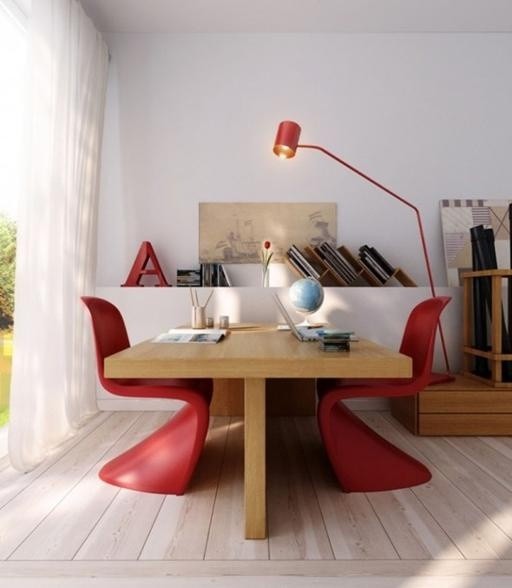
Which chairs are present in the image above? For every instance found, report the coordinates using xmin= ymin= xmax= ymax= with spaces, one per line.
xmin=316 ymin=294 xmax=452 ymax=494
xmin=80 ymin=295 xmax=213 ymax=498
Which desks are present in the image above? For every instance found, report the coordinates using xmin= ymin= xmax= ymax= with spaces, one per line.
xmin=103 ymin=320 xmax=413 ymax=541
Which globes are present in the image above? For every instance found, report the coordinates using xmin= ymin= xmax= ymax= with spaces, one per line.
xmin=289 ymin=276 xmax=324 ymax=327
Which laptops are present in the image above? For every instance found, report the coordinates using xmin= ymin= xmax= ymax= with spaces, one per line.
xmin=273 ymin=292 xmax=358 ymax=343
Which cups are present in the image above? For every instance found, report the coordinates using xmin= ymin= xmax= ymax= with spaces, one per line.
xmin=192 ymin=306 xmax=214 ymax=328
xmin=219 ymin=315 xmax=229 ymax=329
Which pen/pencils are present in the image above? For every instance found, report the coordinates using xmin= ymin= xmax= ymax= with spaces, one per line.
xmin=189 ymin=287 xmax=214 ymax=308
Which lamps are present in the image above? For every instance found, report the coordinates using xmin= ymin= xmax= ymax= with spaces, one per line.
xmin=272 ymin=119 xmax=451 ymax=374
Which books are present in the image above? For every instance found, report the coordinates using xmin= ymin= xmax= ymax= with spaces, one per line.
xmin=150 ymin=327 xmax=232 ymax=345
xmin=176 ymin=262 xmax=231 ymax=286
xmin=287 ymin=241 xmax=394 ymax=284
